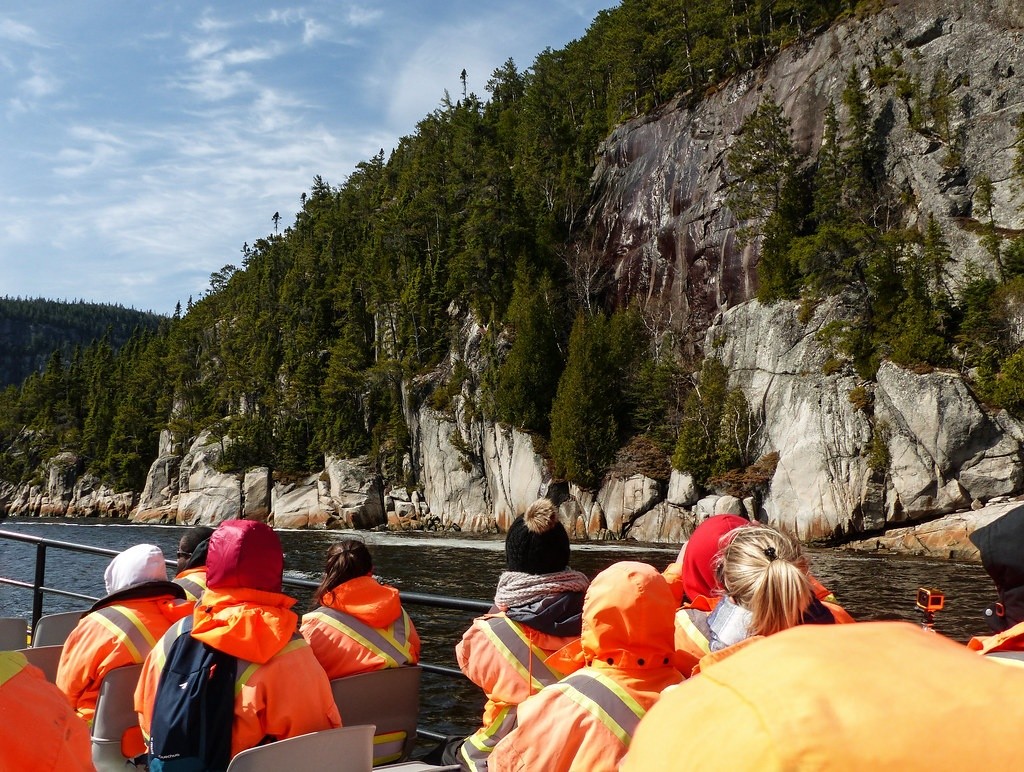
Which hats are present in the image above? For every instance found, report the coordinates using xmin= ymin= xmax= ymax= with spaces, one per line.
xmin=506 ymin=498 xmax=570 ymax=573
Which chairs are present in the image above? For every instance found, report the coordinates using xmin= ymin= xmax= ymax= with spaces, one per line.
xmin=0 ymin=617 xmax=28 ymax=651
xmin=15 ymin=644 xmax=64 ymax=683
xmin=330 ymin=666 xmax=423 ymax=763
xmin=31 ymin=609 xmax=89 ymax=648
xmin=90 ymin=663 xmax=147 ymax=772
xmin=226 ymin=724 xmax=377 ymax=772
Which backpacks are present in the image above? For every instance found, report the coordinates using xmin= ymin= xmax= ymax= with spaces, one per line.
xmin=133 ymin=632 xmax=237 ymax=772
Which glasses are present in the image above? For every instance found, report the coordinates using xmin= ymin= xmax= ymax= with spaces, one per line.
xmin=176 ymin=549 xmax=191 ymax=558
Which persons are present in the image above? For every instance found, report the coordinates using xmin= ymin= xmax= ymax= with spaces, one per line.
xmin=298 ymin=539 xmax=420 ymax=769
xmin=617 ymin=622 xmax=1024 ymax=772
xmin=421 ymin=497 xmax=590 ymax=772
xmin=661 ymin=514 xmax=856 ymax=671
xmin=964 ymin=505 xmax=1024 ymax=656
xmin=171 ymin=526 xmax=214 ymax=604
xmin=487 ymin=561 xmax=688 ymax=772
xmin=123 ymin=520 xmax=342 ymax=772
xmin=55 ymin=543 xmax=194 ymax=733
